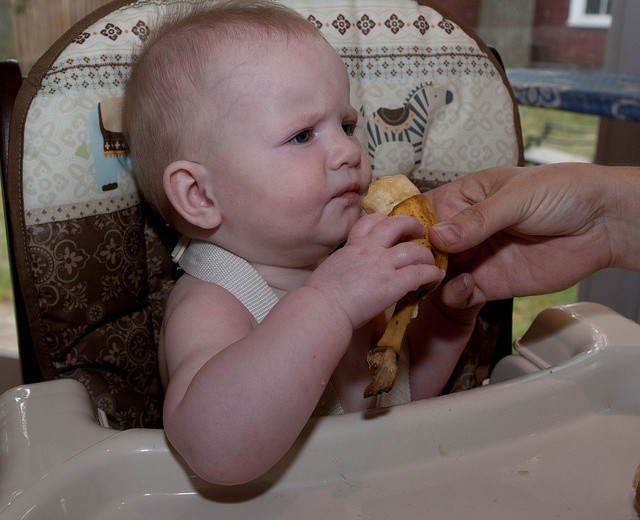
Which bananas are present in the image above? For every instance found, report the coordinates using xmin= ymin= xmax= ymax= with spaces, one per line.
xmin=361 ymin=174 xmax=448 ymax=400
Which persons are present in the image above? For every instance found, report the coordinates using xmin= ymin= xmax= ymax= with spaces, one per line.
xmin=123 ymin=4 xmax=529 ymax=486
xmin=425 ymin=162 xmax=640 ymax=312
